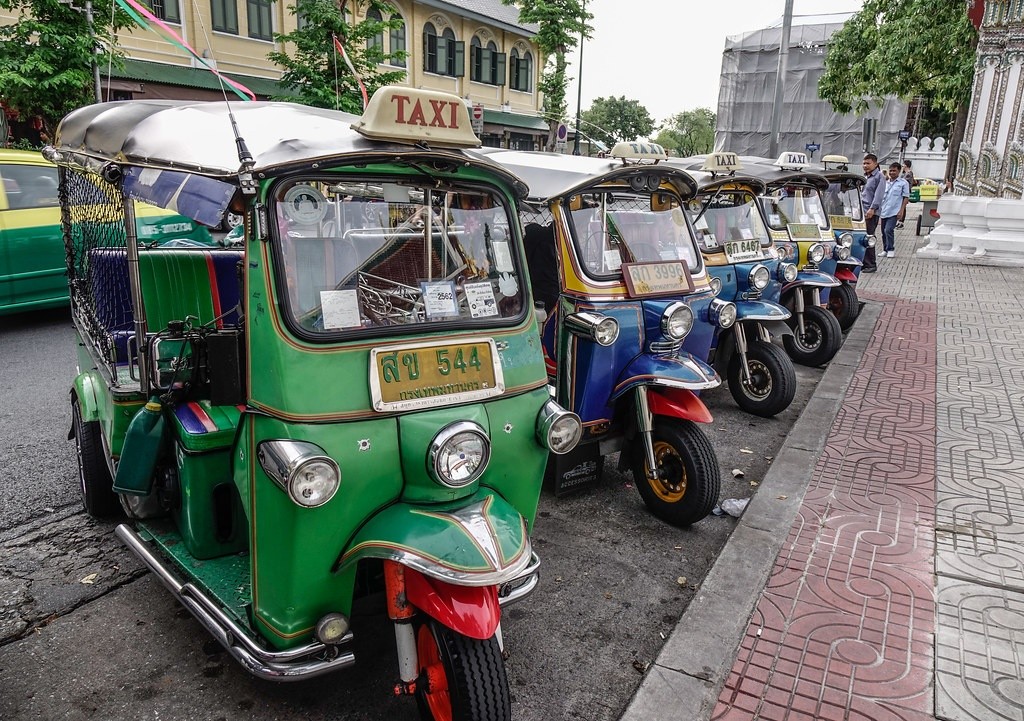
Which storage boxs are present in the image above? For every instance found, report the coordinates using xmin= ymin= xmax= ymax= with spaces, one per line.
xmin=911 ymin=185 xmax=939 ymax=199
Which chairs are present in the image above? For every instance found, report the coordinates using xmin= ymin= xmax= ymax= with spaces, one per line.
xmin=15 ymin=176 xmax=60 ymax=207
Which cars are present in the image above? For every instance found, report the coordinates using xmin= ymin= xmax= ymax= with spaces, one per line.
xmin=0 ymin=147 xmax=214 ymax=319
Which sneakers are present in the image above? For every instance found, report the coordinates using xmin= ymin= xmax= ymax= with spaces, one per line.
xmin=887 ymin=251 xmax=894 ymax=258
xmin=861 ymin=267 xmax=877 ymax=273
xmin=878 ymin=251 xmax=887 ymax=256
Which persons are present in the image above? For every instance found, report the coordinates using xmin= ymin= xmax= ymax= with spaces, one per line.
xmin=860 ymin=154 xmax=913 ymax=273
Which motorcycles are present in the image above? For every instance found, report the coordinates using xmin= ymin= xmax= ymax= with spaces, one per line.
xmin=460 ymin=140 xmax=879 ymax=531
xmin=41 ymin=84 xmax=585 ymax=721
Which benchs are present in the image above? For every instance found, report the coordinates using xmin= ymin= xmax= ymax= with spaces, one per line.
xmin=591 ymin=209 xmax=676 ymax=242
xmin=87 ymin=245 xmax=246 ymax=452
xmin=343 ymin=227 xmax=506 ymax=272
